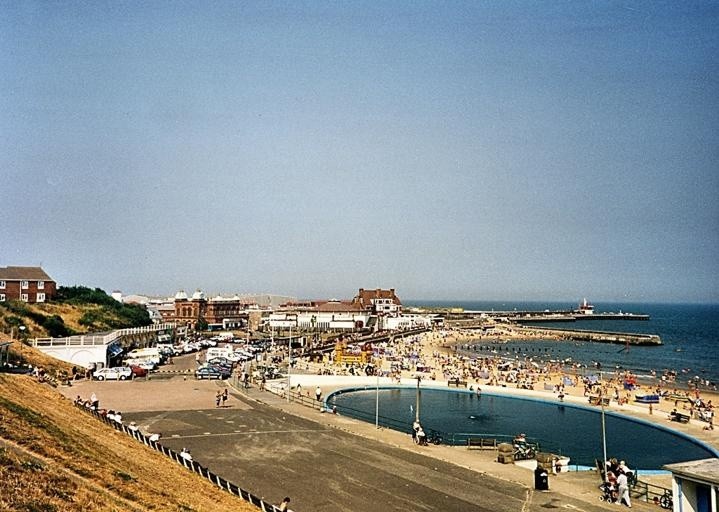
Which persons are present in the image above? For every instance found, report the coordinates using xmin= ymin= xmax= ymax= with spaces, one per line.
xmin=74 ymin=391 xmax=194 ymax=471
xmin=30 ymin=363 xmax=98 ymax=386
xmin=281 ymin=496 xmax=293 ymax=511
xmin=598 ymin=456 xmax=634 ymax=507
xmin=195 ymin=326 xmax=719 ymax=458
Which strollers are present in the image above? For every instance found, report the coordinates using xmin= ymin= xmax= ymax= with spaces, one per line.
xmin=599 ymin=470 xmax=620 ymax=503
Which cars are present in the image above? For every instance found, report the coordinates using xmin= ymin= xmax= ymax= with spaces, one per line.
xmin=89 ymin=331 xmax=272 ymax=382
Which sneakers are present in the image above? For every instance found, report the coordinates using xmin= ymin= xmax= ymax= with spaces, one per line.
xmin=628 ymin=503 xmax=632 ymax=508
xmin=614 ymin=501 xmax=622 ymax=506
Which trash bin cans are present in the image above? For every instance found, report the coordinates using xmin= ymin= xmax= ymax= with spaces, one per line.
xmin=535 ymin=465 xmax=548 ymax=490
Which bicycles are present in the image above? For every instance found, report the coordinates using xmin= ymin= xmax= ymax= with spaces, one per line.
xmin=660 ymin=489 xmax=672 ymax=508
xmin=426 ymin=430 xmax=443 ymax=445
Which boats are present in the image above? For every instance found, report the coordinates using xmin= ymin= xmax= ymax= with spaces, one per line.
xmin=665 ymin=394 xmax=690 ymax=403
xmin=634 ymin=393 xmax=660 ymax=403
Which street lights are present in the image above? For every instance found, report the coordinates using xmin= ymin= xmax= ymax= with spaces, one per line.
xmin=412 ymin=371 xmax=426 ymax=425
xmin=589 ymin=371 xmax=608 ymax=478
xmin=19 ymin=326 xmax=26 ymax=362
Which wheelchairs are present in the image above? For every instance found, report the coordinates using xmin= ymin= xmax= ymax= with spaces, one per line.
xmin=416 ymin=430 xmax=427 ymax=446
